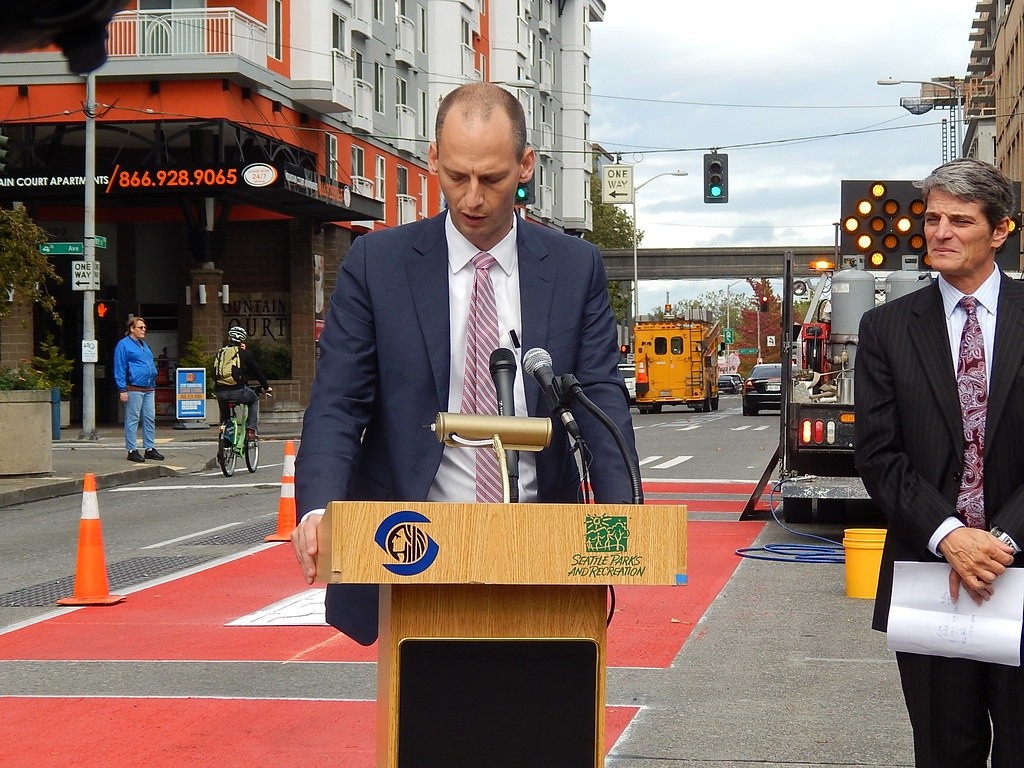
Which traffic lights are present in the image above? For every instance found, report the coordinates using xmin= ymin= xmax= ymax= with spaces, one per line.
xmin=515 ymin=171 xmax=535 ymax=205
xmin=704 ymin=153 xmax=728 ymax=204
xmin=665 ymin=304 xmax=672 ymax=316
xmin=759 ymin=294 xmax=769 ymax=314
xmin=94 ymin=300 xmax=110 ymax=323
xmin=621 ymin=345 xmax=630 ymax=354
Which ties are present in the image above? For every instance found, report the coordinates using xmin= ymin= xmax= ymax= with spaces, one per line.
xmin=460 ymin=253 xmax=504 ymax=503
xmin=956 ymin=296 xmax=987 ymax=530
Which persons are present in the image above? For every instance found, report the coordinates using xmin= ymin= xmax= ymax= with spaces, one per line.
xmin=854 ymin=159 xmax=1023 ymax=768
xmin=212 ymin=327 xmax=273 ymax=466
xmin=114 ymin=317 xmax=164 ymax=462
xmin=291 ymin=82 xmax=645 ymax=646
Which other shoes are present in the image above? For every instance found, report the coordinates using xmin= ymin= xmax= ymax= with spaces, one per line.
xmin=224 ymin=438 xmax=232 ymax=448
xmin=247 ymin=429 xmax=258 ymax=441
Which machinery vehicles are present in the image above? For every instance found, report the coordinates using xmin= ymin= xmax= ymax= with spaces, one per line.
xmin=633 ymin=308 xmax=725 ymax=414
xmin=779 ymin=181 xmax=1023 ymax=525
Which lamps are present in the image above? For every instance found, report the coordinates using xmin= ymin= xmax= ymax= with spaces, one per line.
xmin=199 ymin=285 xmax=209 ymax=304
xmin=218 ymin=284 xmax=230 ymax=304
xmin=186 ymin=286 xmax=192 ymax=305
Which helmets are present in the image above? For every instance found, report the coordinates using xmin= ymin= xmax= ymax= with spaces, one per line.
xmin=228 ymin=326 xmax=247 ymax=342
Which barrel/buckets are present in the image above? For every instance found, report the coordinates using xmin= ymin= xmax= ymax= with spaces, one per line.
xmin=842 ymin=528 xmax=887 ymax=599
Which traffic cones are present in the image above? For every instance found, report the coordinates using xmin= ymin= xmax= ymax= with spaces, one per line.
xmin=56 ymin=473 xmax=126 ymax=604
xmin=263 ymin=440 xmax=297 ymax=542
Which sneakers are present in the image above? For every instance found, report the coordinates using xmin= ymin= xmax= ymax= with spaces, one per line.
xmin=127 ymin=449 xmax=145 ymax=462
xmin=144 ymin=448 xmax=164 ymax=460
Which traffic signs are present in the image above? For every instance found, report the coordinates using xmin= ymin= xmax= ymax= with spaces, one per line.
xmin=73 ymin=262 xmax=100 ymax=290
xmin=601 ymin=165 xmax=633 ymax=204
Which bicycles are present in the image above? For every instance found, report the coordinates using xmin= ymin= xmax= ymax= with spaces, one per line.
xmin=217 ymin=385 xmax=273 ymax=477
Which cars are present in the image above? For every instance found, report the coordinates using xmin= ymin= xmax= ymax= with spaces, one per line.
xmin=718 ymin=374 xmax=745 ymax=395
xmin=742 ymin=364 xmax=796 ymax=416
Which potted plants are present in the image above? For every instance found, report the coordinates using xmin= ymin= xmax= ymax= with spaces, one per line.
xmin=174 ymin=335 xmax=221 ymax=425
xmin=27 ymin=336 xmax=72 ymax=426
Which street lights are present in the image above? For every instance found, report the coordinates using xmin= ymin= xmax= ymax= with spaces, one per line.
xmin=633 ymin=171 xmax=688 ymax=325
xmin=877 ymin=77 xmax=962 ymax=160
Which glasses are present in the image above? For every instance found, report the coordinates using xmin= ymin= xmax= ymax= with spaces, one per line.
xmin=135 ymin=326 xmax=147 ymax=329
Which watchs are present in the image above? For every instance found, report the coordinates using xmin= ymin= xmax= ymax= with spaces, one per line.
xmin=990 ymin=526 xmax=1017 ymax=555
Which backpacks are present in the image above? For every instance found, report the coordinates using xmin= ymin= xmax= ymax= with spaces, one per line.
xmin=213 ymin=346 xmax=242 ymax=385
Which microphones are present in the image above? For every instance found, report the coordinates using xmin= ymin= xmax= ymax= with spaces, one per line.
xmin=489 ymin=348 xmax=519 ymax=502
xmin=522 ymin=347 xmax=581 ymax=440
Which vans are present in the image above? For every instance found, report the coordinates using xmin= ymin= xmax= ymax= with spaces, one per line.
xmin=618 ymin=364 xmax=636 ymax=405
